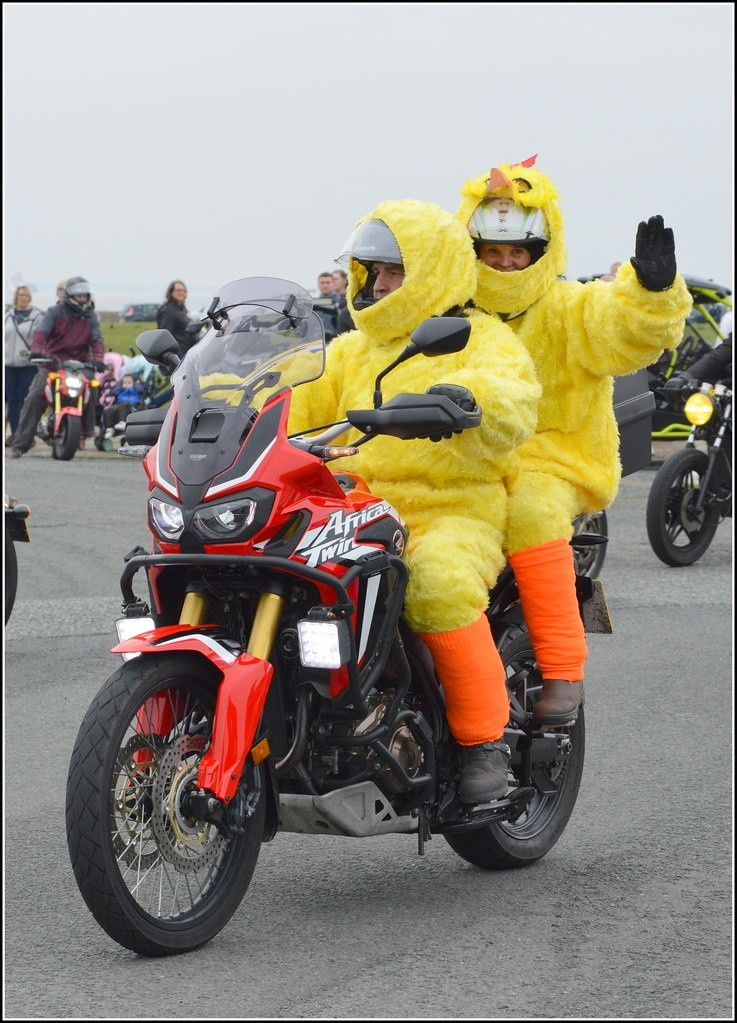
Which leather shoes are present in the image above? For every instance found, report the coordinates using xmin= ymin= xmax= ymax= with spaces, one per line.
xmin=533 ymin=676 xmax=586 ymax=724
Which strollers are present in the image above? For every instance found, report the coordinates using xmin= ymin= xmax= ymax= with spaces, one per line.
xmin=79 ymin=346 xmax=161 ymax=452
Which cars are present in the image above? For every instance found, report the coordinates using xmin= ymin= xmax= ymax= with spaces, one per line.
xmin=121 ymin=302 xmax=163 ymax=322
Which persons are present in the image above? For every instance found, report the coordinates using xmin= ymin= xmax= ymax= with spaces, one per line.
xmin=314 ymin=269 xmax=350 ymax=343
xmin=5 ymin=276 xmax=200 ymax=459
xmin=197 ymin=199 xmax=544 ymax=803
xmin=666 ymin=332 xmax=733 ymax=404
xmin=455 ymin=154 xmax=693 ymax=722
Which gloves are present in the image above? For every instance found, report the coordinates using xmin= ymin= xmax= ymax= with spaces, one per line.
xmin=28 ymin=352 xmax=43 ymax=363
xmin=425 ymin=384 xmax=477 ymax=443
xmin=630 ymin=214 xmax=677 ymax=291
xmin=92 ymin=361 xmax=107 ymax=373
xmin=664 ymin=377 xmax=686 ymax=394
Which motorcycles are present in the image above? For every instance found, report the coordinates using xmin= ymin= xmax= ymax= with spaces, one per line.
xmin=64 ymin=277 xmax=732 ymax=956
xmin=31 ymin=354 xmax=111 ymax=460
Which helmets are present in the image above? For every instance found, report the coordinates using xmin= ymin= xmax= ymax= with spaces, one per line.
xmin=466 ymin=198 xmax=550 ymax=322
xmin=335 ymin=218 xmax=404 ymax=311
xmin=65 ymin=276 xmax=91 ymax=312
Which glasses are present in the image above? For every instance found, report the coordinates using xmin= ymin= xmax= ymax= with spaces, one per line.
xmin=174 ymin=289 xmax=187 ymax=293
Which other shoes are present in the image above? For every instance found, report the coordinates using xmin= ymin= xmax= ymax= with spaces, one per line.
xmin=458 ymin=735 xmax=512 ymax=805
xmin=5 ymin=434 xmax=17 ymax=447
xmin=114 ymin=421 xmax=126 ymax=431
xmin=105 ymin=428 xmax=115 ymax=439
xmin=79 ymin=437 xmax=86 ymax=451
xmin=9 ymin=445 xmax=24 ymax=459
